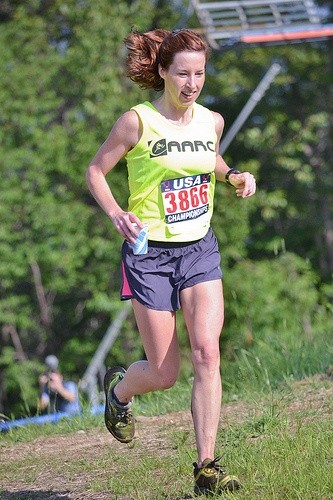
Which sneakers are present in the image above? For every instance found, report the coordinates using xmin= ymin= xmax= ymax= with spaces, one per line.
xmin=103 ymin=367 xmax=136 ymax=443
xmin=192 ymin=453 xmax=242 ymax=495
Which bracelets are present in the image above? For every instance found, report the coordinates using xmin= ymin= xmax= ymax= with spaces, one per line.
xmin=225 ymin=167 xmax=240 ymax=187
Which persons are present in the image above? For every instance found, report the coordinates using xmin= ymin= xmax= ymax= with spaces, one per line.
xmin=38 ymin=369 xmax=81 ymax=418
xmin=86 ymin=28 xmax=257 ymax=497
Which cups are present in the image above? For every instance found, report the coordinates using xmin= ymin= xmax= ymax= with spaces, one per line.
xmin=130 ymin=222 xmax=149 ymax=255
